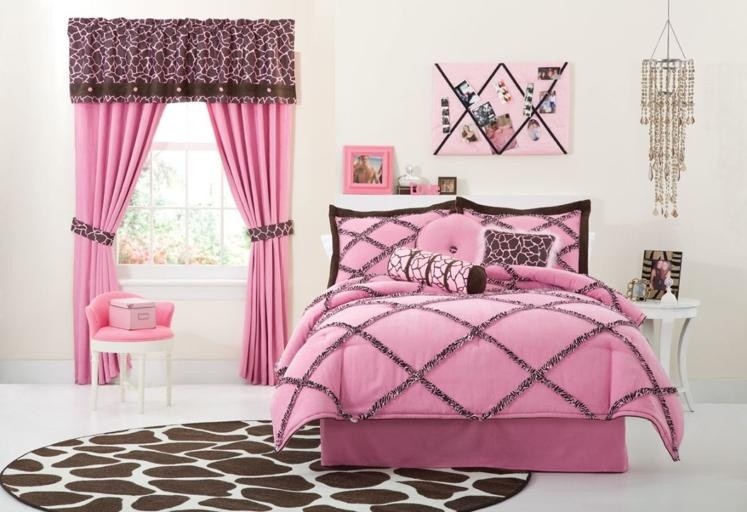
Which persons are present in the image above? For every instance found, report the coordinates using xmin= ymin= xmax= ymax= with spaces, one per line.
xmin=525 ymin=119 xmax=541 ymax=141
xmin=489 ymin=121 xmax=504 ymax=139
xmin=462 ymin=125 xmax=477 ymax=144
xmin=440 ymin=180 xmax=454 ymax=192
xmin=353 ymin=155 xmax=379 ymax=184
xmin=539 ymin=68 xmax=558 ymax=79
xmin=650 ymin=260 xmax=671 ymax=290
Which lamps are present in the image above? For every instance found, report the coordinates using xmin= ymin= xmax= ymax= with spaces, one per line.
xmin=640 ymin=0 xmax=696 ymax=220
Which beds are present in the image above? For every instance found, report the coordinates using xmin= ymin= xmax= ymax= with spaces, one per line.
xmin=318 ymin=194 xmax=628 ymax=472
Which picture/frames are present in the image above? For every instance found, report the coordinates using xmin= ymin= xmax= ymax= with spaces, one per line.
xmin=639 ymin=248 xmax=683 ymax=303
xmin=339 ymin=144 xmax=393 ymax=194
xmin=437 ymin=176 xmax=458 ymax=195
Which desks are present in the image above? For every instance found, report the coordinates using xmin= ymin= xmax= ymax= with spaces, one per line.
xmin=631 ymin=298 xmax=701 ymax=414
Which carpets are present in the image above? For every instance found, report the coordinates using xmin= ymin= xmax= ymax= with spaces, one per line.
xmin=0 ymin=415 xmax=532 ymax=511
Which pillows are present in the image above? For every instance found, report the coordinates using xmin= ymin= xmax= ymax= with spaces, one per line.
xmin=320 ymin=195 xmax=591 ymax=294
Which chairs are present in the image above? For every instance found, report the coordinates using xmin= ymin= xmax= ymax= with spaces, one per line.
xmin=83 ymin=290 xmax=175 ymax=420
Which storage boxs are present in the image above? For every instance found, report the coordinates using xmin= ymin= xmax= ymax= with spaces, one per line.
xmin=107 ymin=298 xmax=157 ymax=330
